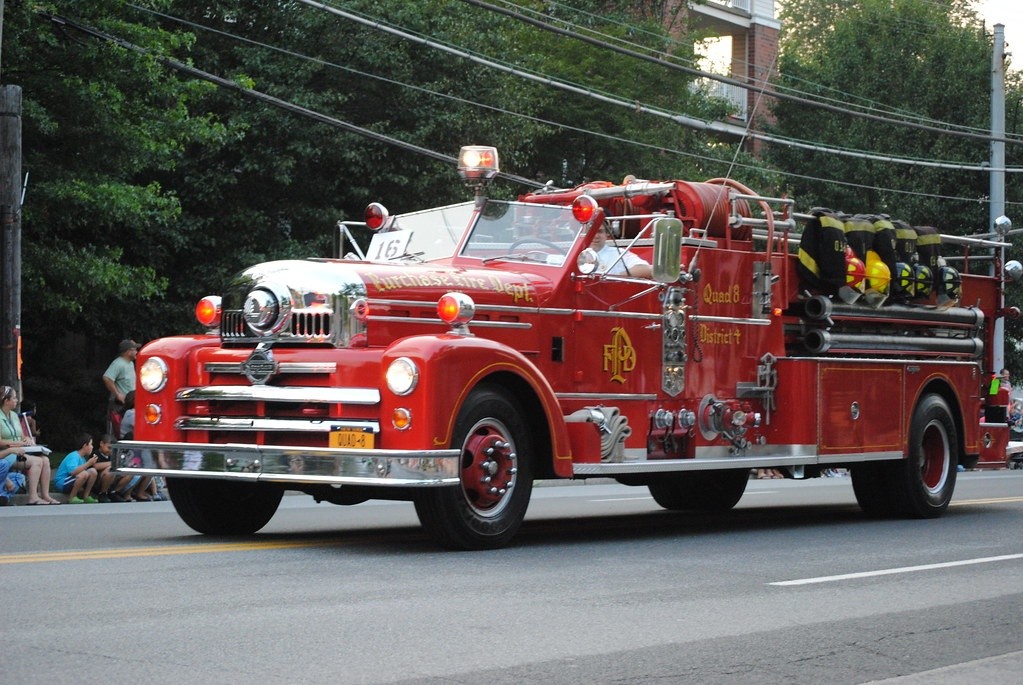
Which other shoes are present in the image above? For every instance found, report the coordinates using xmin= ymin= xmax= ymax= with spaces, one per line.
xmin=0 ymin=496 xmax=8 ymax=506
xmin=136 ymin=494 xmax=151 ymax=502
xmin=152 ymin=492 xmax=162 ymax=500
xmin=95 ymin=491 xmax=111 ymax=503
xmin=107 ymin=490 xmax=124 ymax=502
xmin=122 ymin=494 xmax=133 ymax=502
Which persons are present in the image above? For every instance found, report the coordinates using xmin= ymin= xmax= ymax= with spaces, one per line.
xmin=589 ymin=220 xmax=653 ymax=280
xmin=0 ymin=338 xmax=173 ymax=504
xmin=1000 ymin=368 xmax=1010 ymax=419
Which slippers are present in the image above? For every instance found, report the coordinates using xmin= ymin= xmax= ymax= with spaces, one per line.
xmin=25 ymin=498 xmax=51 ymax=505
xmin=47 ymin=497 xmax=61 ymax=505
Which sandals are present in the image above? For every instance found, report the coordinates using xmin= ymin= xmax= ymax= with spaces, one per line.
xmin=83 ymin=495 xmax=98 ymax=503
xmin=68 ymin=496 xmax=84 ymax=504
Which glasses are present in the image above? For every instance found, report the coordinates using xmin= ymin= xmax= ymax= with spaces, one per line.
xmin=128 ymin=348 xmax=138 ymax=352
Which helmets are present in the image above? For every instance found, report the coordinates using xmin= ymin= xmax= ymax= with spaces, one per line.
xmin=866 ymin=250 xmax=892 ymax=292
xmin=934 ymin=266 xmax=961 ymax=298
xmin=913 ymin=262 xmax=934 ymax=301
xmin=845 ymin=245 xmax=867 ymax=287
xmin=894 ymin=262 xmax=915 ymax=301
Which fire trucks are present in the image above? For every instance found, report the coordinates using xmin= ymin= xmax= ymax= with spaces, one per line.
xmin=110 ymin=143 xmax=1022 ymax=551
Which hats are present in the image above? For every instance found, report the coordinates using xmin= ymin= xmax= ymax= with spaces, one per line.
xmin=118 ymin=339 xmax=142 ymax=352
xmin=602 ymin=208 xmax=615 ymax=222
